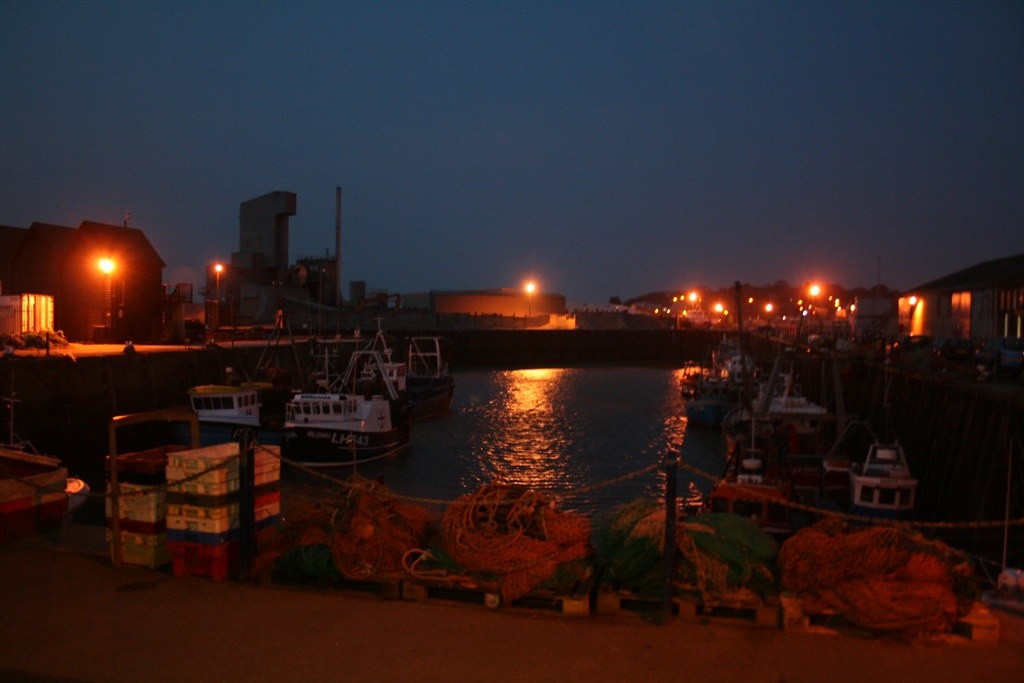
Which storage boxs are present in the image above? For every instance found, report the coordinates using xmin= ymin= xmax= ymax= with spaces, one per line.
xmin=162 ymin=493 xmax=283 ymax=536
xmin=104 ymin=484 xmax=166 ymax=525
xmin=105 ymin=440 xmax=192 ymax=487
xmin=166 ymin=525 xmax=281 ymax=579
xmin=104 ymin=529 xmax=166 ymax=569
xmin=163 ymin=443 xmax=281 ymax=497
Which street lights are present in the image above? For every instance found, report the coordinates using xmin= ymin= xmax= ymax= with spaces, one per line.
xmin=215 ymin=263 xmax=226 ymax=298
xmin=523 ymin=280 xmax=536 ymax=317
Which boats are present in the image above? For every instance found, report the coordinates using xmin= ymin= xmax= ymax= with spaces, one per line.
xmin=0 ymin=302 xmax=457 ymax=543
xmin=681 ymin=281 xmax=919 ymax=544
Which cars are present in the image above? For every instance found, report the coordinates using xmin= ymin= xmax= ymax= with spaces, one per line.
xmin=926 ymin=335 xmax=977 ymax=372
xmin=984 ymin=336 xmax=1024 ymax=379
